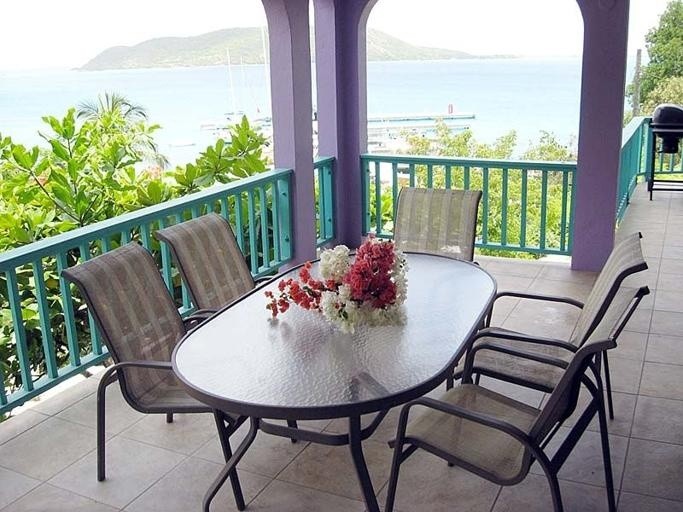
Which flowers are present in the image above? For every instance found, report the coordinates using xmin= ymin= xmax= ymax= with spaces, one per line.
xmin=264 ymin=233 xmax=408 ymax=335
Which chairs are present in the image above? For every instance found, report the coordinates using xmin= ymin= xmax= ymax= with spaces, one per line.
xmin=446 ymin=230 xmax=650 ymax=468
xmin=151 ymin=209 xmax=301 ymax=443
xmin=384 ymin=285 xmax=650 ymax=512
xmin=60 ymin=240 xmax=301 ymax=511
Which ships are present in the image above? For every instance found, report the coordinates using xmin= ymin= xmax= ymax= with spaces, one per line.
xmin=196 ymin=104 xmax=474 ymax=177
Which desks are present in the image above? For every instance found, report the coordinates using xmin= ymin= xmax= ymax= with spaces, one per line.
xmin=171 ymin=250 xmax=496 ymax=511
xmin=392 ymin=186 xmax=485 ymax=394
